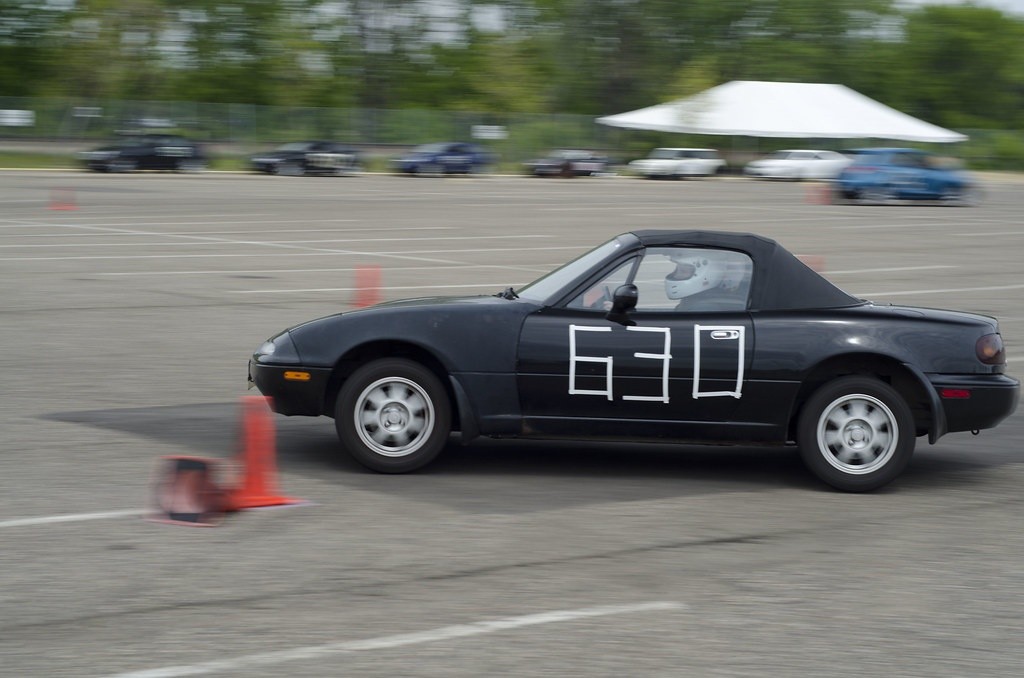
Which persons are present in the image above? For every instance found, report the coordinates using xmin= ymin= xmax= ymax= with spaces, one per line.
xmin=601 ymin=247 xmax=738 ymax=312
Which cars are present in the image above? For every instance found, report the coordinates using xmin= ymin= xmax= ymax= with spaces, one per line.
xmin=632 ymin=146 xmax=730 ymax=179
xmin=252 ymin=140 xmax=364 ymax=174
xmin=534 ymin=145 xmax=605 ymax=178
xmin=389 ymin=141 xmax=497 ymax=175
xmin=835 ymin=147 xmax=961 ymax=202
xmin=80 ymin=131 xmax=201 ymax=174
xmin=742 ymin=149 xmax=852 ymax=183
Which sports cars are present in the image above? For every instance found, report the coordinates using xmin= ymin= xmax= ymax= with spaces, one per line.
xmin=247 ymin=229 xmax=1021 ymax=493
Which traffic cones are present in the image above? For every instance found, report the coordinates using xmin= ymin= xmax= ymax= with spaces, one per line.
xmin=217 ymin=394 xmax=291 ymax=512
xmin=148 ymin=451 xmax=234 ymax=529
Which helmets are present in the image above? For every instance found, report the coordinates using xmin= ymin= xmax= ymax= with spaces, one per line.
xmin=665 ymin=248 xmax=727 ymax=300
